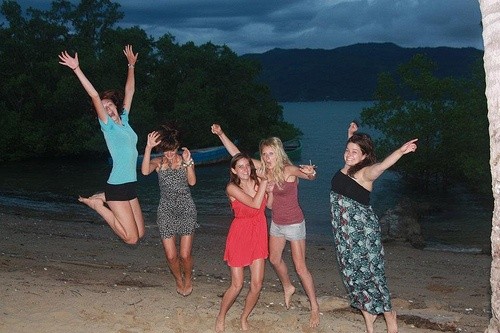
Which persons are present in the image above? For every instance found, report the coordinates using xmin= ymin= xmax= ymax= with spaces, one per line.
xmin=58 ymin=44 xmax=145 ymax=246
xmin=142 ymin=131 xmax=201 ymax=296
xmin=329 ymin=121 xmax=419 ymax=333
xmin=215 ymin=153 xmax=275 ymax=333
xmin=211 ymin=124 xmax=321 ymax=328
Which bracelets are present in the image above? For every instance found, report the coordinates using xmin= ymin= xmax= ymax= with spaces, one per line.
xmin=217 ymin=131 xmax=224 ymax=136
xmin=73 ymin=65 xmax=79 ymax=70
xmin=182 ymin=160 xmax=194 ymax=167
xmin=311 ymin=171 xmax=317 ymax=177
xmin=127 ymin=63 xmax=135 ymax=67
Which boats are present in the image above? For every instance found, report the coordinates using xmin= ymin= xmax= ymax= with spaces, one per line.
xmin=107 ymin=139 xmax=239 ymax=172
xmin=254 ymin=135 xmax=303 ymax=162
